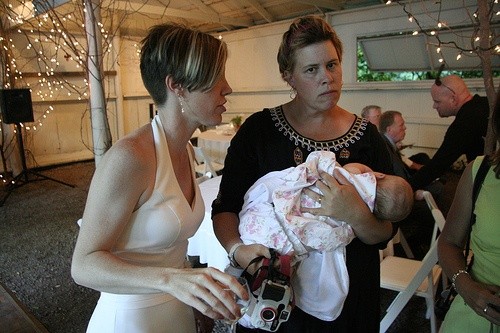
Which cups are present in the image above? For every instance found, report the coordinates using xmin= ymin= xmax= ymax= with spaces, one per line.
xmin=201 ymin=276 xmax=251 ymax=333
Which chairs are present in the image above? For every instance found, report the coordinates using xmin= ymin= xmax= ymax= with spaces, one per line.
xmin=380 ymin=208 xmax=445 ymax=333
xmin=192 ymin=146 xmax=217 ymax=184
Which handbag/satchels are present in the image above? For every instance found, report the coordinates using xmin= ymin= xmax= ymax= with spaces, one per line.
xmin=434 ymin=153 xmax=494 ymax=320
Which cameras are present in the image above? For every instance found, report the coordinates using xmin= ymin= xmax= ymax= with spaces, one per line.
xmin=250 ymin=278 xmax=293 ymax=333
xmin=487 ymin=291 xmax=500 ymax=314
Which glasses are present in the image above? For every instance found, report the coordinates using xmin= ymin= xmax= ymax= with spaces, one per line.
xmin=369 ymin=114 xmax=384 ymax=118
xmin=435 ymin=80 xmax=456 ymax=95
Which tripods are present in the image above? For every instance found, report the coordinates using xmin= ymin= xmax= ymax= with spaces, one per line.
xmin=0 ymin=123 xmax=75 ymax=207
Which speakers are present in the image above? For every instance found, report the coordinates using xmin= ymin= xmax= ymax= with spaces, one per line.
xmin=0 ymin=88 xmax=34 ymax=124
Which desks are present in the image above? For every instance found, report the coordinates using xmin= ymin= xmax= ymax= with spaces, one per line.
xmin=199 ymin=120 xmax=245 ymax=165
